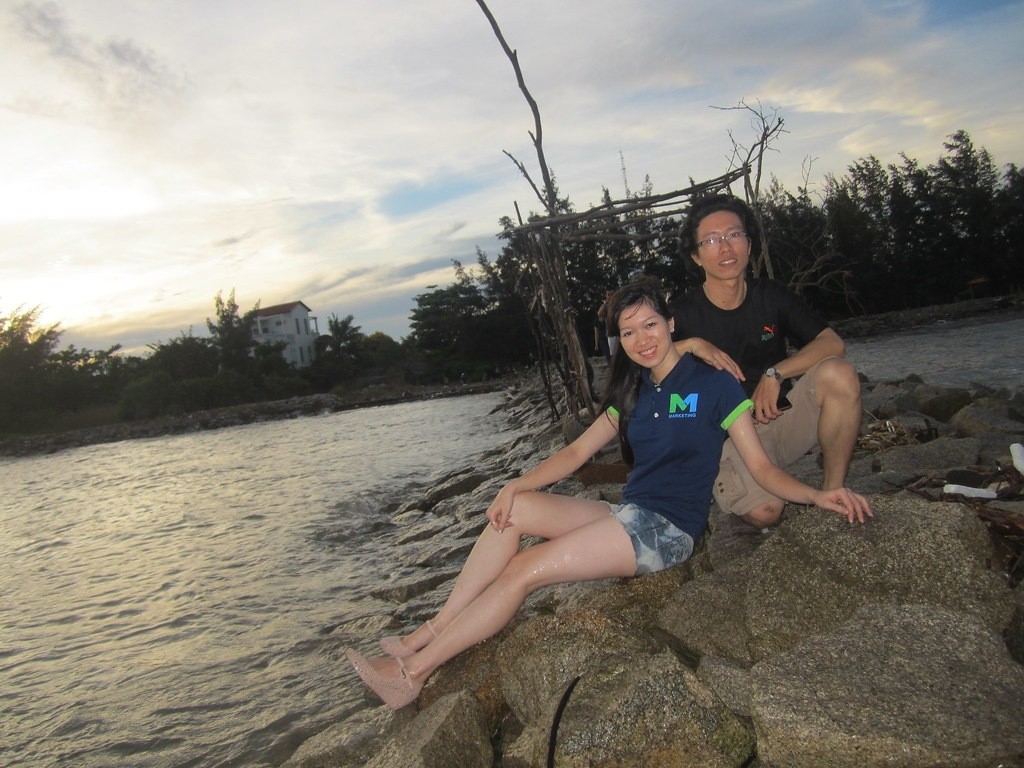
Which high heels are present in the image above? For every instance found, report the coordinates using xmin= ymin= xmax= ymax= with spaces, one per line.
xmin=345 ymin=647 xmax=424 ymax=711
xmin=381 ymin=618 xmax=439 ymax=658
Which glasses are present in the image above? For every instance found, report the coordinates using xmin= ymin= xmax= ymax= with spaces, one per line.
xmin=687 ymin=230 xmax=749 ymax=250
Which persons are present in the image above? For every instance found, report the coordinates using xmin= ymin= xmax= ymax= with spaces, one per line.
xmin=656 ymin=192 xmax=864 ymax=531
xmin=344 ymin=282 xmax=878 ymax=711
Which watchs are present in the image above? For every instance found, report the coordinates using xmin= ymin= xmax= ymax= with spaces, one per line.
xmin=764 ymin=366 xmax=785 ymax=383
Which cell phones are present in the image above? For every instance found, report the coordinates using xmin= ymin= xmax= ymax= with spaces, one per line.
xmin=750 ymin=394 xmax=792 ymax=419
